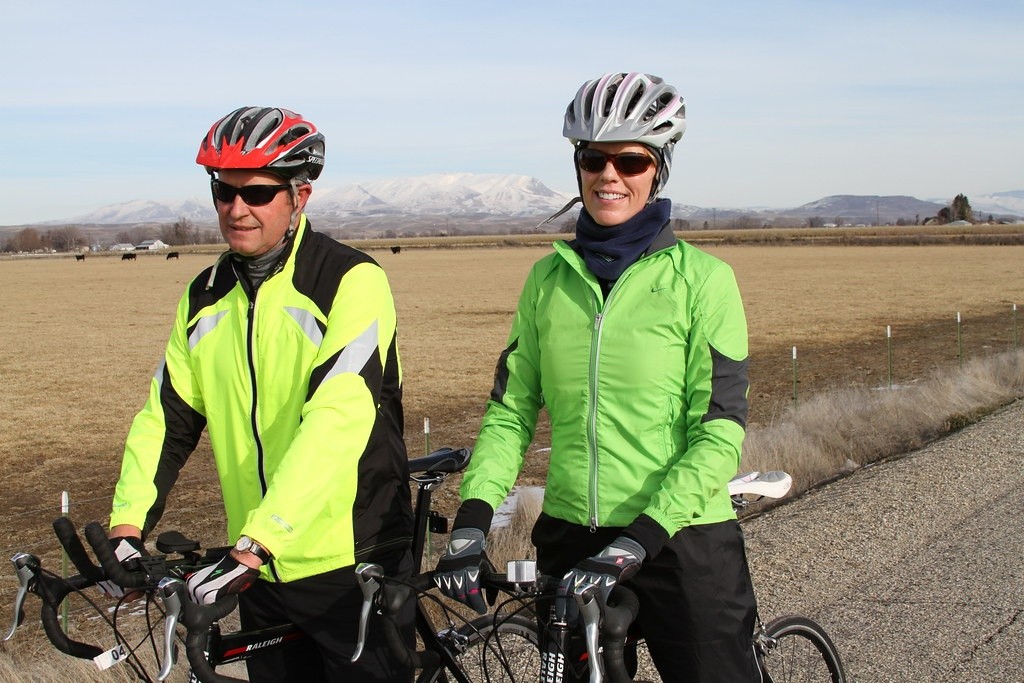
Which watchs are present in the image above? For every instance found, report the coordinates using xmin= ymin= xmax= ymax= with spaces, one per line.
xmin=232 ymin=535 xmax=271 ymax=566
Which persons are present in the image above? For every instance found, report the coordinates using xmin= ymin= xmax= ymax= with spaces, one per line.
xmin=430 ymin=73 xmax=777 ymax=683
xmin=92 ymin=105 xmax=421 ymax=683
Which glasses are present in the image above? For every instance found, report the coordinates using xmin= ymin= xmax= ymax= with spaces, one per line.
xmin=578 ymin=149 xmax=651 ymax=176
xmin=211 ymin=179 xmax=292 ymax=206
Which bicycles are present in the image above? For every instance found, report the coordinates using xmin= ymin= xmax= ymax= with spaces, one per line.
xmin=351 ymin=471 xmax=847 ymax=683
xmin=4 ymin=447 xmax=542 ymax=683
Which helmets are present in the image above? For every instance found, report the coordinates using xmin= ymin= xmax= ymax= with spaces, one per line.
xmin=195 ymin=106 xmax=325 ymax=181
xmin=562 ymin=72 xmax=686 ymax=146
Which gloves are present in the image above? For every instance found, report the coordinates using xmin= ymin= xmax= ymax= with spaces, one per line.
xmin=555 ymin=537 xmax=643 ymax=622
xmin=434 ymin=529 xmax=498 ymax=615
xmin=185 ymin=555 xmax=260 ymax=605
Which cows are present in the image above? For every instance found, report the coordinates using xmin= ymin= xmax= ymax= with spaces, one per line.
xmin=121 ymin=253 xmax=137 ymax=261
xmin=390 ymin=246 xmax=401 ymax=254
xmin=166 ymin=252 xmax=179 ymax=260
xmin=75 ymin=255 xmax=85 ymax=261
